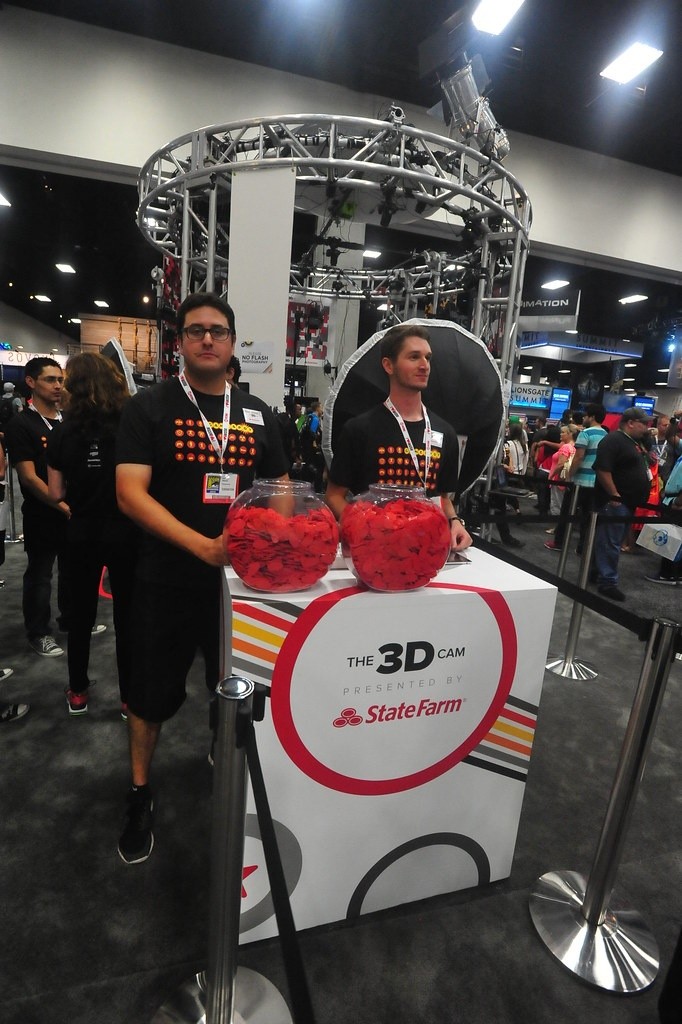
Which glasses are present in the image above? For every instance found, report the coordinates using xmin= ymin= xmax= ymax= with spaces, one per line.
xmin=35 ymin=375 xmax=67 ymax=387
xmin=177 ymin=324 xmax=235 ymax=342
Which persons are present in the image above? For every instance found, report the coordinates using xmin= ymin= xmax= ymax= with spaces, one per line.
xmin=0 ymin=353 xmax=132 ymax=722
xmin=287 ymin=402 xmax=327 ymax=495
xmin=116 ymin=292 xmax=296 ymax=865
xmin=324 ymin=325 xmax=473 ymax=552
xmin=479 ymin=404 xmax=682 ymax=603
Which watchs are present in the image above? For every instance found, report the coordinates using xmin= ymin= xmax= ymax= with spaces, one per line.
xmin=449 ymin=517 xmax=466 ymax=526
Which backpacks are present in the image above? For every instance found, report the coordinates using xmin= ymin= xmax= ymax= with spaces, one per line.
xmin=0 ymin=396 xmax=19 ymax=425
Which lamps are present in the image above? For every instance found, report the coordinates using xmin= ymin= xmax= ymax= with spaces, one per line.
xmin=33 ymin=287 xmax=54 ymax=303
xmin=71 ymin=314 xmax=81 ymax=324
xmin=93 ymin=295 xmax=112 ymax=308
xmin=363 ymin=226 xmax=383 ymax=259
xmin=417 ymin=0 xmax=531 ymax=100
xmin=55 ymin=254 xmax=80 ymax=274
xmin=558 ymin=365 xmax=571 ymax=374
xmin=535 ymin=261 xmax=574 ymax=292
xmin=0 ymin=180 xmax=18 ymax=208
xmin=617 ymin=280 xmax=649 ymax=305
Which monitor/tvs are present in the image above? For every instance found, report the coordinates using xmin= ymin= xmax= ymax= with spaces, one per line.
xmin=632 ymin=397 xmax=655 ymax=415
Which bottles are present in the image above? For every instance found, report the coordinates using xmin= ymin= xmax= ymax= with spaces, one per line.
xmin=338 ymin=482 xmax=451 ymax=591
xmin=221 ymin=478 xmax=338 ymax=592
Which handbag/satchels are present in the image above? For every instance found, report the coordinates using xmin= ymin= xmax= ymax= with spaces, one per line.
xmin=559 ymin=451 xmax=577 ymax=481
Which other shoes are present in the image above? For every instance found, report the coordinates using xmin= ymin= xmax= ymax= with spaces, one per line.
xmin=314 ymin=489 xmax=325 ymax=495
xmin=673 ymin=576 xmax=682 ymax=585
xmin=544 ymin=541 xmax=564 ymax=552
xmin=573 ymin=548 xmax=582 ymax=557
xmin=619 ymin=545 xmax=632 ymax=552
xmin=544 ymin=527 xmax=556 ymax=536
xmin=502 ymin=533 xmax=526 ymax=547
xmin=644 ymin=572 xmax=677 ymax=585
xmin=604 ymin=585 xmax=626 ymax=602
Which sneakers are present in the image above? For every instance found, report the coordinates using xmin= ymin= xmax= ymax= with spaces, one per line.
xmin=0 ymin=702 xmax=31 ymax=725
xmin=0 ymin=667 xmax=15 ymax=681
xmin=28 ymin=634 xmax=64 ymax=657
xmin=64 ymin=685 xmax=88 ymax=717
xmin=57 ymin=617 xmax=107 ymax=637
xmin=120 ymin=706 xmax=130 ymax=720
xmin=208 ymin=734 xmax=215 ymax=765
xmin=116 ymin=791 xmax=156 ymax=864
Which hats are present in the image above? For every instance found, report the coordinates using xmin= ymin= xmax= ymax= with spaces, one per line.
xmin=3 ymin=381 xmax=16 ymax=391
xmin=621 ymin=406 xmax=657 ymax=424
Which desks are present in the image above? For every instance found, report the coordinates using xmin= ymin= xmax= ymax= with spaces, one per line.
xmin=214 ymin=539 xmax=557 ymax=946
xmin=472 ymin=474 xmax=498 ymax=508
